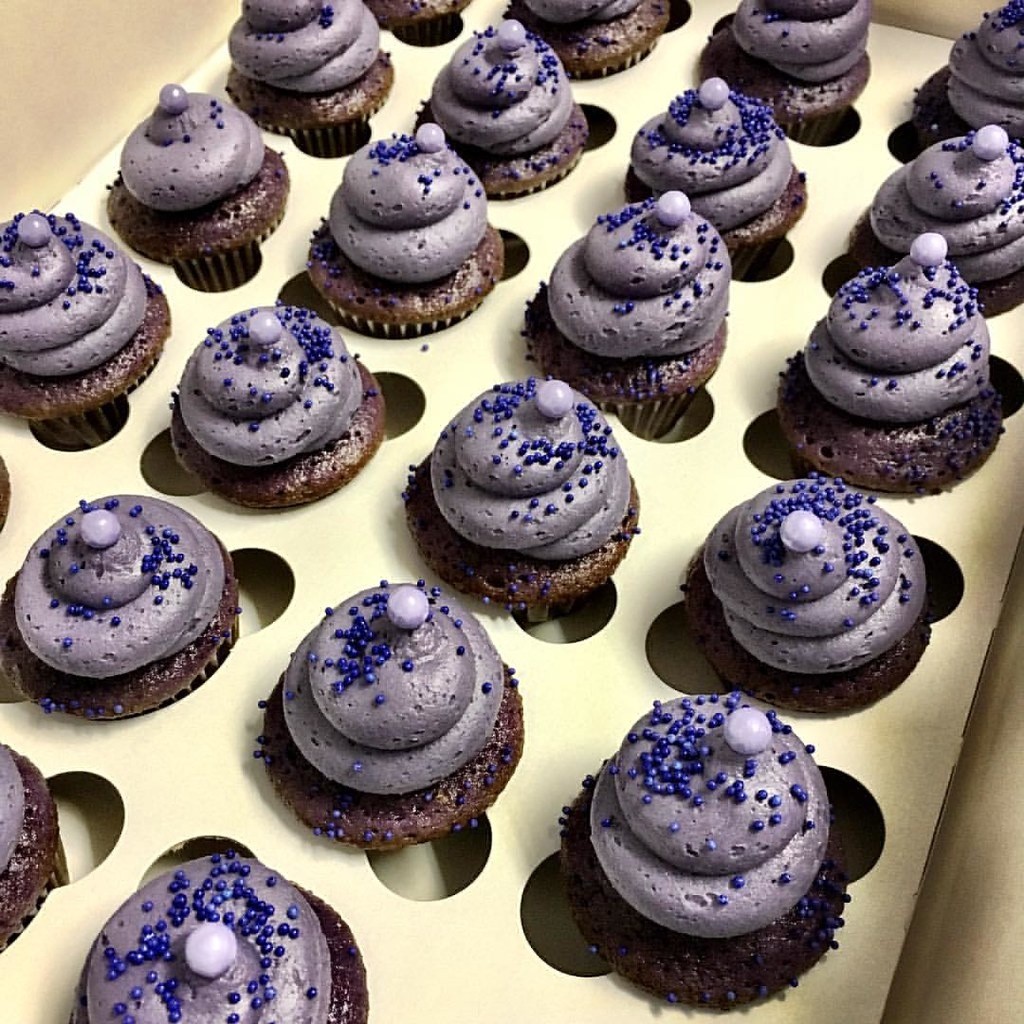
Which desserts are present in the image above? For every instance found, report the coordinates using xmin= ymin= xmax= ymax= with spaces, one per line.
xmin=0 ymin=1 xmax=1024 ymax=1024
